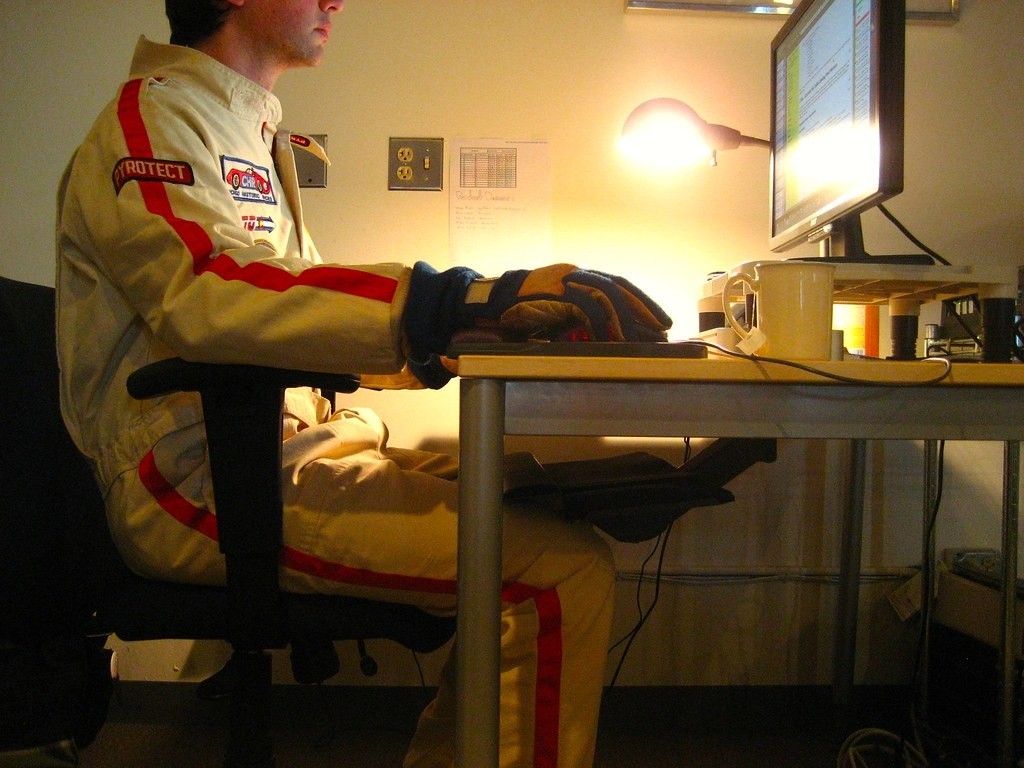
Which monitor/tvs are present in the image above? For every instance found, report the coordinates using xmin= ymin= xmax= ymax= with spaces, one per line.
xmin=769 ymin=0 xmax=936 ymax=267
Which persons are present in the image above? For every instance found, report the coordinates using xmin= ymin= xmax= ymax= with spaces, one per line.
xmin=54 ymin=1 xmax=674 ymax=767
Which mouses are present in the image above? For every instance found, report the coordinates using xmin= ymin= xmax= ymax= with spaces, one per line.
xmin=528 ymin=306 xmax=667 ymax=343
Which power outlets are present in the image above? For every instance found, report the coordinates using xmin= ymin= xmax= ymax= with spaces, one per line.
xmin=387 ymin=136 xmax=444 ymax=191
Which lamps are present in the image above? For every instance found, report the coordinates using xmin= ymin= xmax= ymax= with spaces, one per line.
xmin=620 ymin=96 xmax=769 ymax=168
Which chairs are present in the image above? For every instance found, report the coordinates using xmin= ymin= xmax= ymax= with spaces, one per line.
xmin=0 ymin=275 xmax=457 ymax=768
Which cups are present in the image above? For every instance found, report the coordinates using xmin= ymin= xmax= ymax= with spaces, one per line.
xmin=721 ymin=262 xmax=836 ymax=360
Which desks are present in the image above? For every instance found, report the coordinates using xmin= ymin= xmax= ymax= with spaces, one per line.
xmin=452 ymin=354 xmax=1024 ymax=768
xmin=696 ymin=260 xmax=1018 ymax=363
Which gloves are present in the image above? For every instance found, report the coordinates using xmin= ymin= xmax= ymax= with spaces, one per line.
xmin=461 ymin=263 xmax=673 ymax=341
